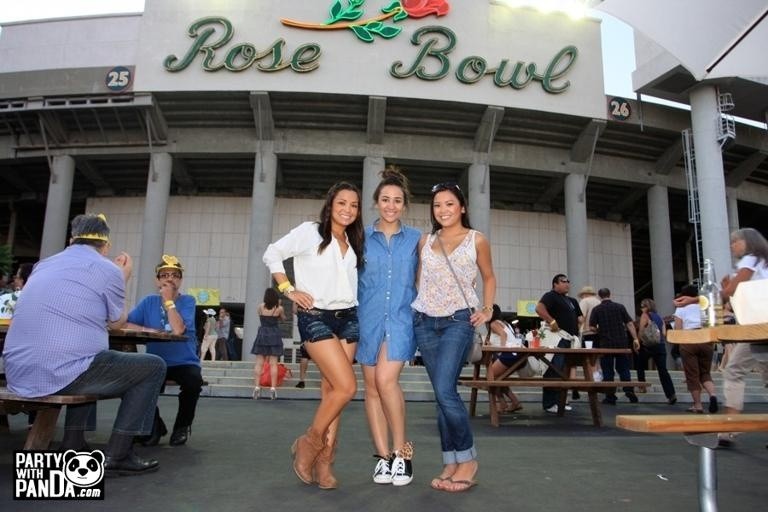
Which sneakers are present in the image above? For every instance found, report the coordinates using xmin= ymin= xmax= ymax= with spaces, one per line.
xmin=390 ymin=440 xmax=416 ymax=486
xmin=369 ymin=451 xmax=395 ymax=486
xmin=499 ymin=384 xmax=736 ymax=447
xmin=296 ymin=382 xmax=305 ymax=388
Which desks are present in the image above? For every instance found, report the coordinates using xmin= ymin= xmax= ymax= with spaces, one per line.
xmin=665 ymin=323 xmax=768 ymax=377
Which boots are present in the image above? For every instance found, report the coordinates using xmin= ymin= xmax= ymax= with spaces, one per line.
xmin=315 ymin=438 xmax=340 ymax=489
xmin=290 ymin=426 xmax=324 ymax=486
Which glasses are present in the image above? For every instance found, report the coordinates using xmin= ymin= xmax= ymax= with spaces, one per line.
xmin=430 ymin=181 xmax=457 ymax=192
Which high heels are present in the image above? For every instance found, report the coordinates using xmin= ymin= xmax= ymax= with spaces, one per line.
xmin=270 ymin=387 xmax=277 ymax=401
xmin=252 ymin=386 xmax=260 ymax=400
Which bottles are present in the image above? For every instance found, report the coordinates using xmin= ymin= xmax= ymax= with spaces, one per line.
xmin=698 ymin=258 xmax=725 ymax=328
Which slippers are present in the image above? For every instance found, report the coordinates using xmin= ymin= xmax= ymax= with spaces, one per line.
xmin=444 ymin=478 xmax=479 ymax=493
xmin=430 ymin=476 xmax=449 ymax=491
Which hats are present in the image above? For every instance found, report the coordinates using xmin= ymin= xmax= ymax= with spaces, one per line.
xmin=203 ymin=308 xmax=216 ymax=316
xmin=579 ymin=286 xmax=596 ymax=299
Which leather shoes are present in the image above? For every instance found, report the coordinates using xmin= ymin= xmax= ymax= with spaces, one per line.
xmin=170 ymin=427 xmax=187 ymax=445
xmin=104 ymin=449 xmax=161 ymax=479
xmin=141 ymin=417 xmax=168 ymax=446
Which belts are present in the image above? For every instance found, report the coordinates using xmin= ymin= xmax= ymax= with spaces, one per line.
xmin=297 ymin=305 xmax=356 ymax=318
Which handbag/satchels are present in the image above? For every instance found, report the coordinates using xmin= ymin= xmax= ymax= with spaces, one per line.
xmin=261 ymin=363 xmax=286 ymax=386
xmin=639 ymin=320 xmax=661 ymax=349
xmin=465 ymin=332 xmax=483 ymax=364
xmin=540 ymin=323 xmax=574 ymax=367
xmin=518 ymin=357 xmax=542 ymax=377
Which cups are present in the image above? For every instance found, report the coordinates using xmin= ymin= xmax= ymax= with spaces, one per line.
xmin=584 ymin=340 xmax=593 ymax=349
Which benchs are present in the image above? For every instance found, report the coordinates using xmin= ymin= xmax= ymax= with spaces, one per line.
xmin=449 ymin=342 xmax=648 ymax=428
xmin=615 ymin=411 xmax=765 ymax=512
xmin=0 ymin=329 xmax=209 ymax=479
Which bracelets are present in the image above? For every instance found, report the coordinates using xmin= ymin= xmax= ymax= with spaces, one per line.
xmin=482 ymin=306 xmax=494 ymax=312
xmin=277 ymin=281 xmax=295 ymax=293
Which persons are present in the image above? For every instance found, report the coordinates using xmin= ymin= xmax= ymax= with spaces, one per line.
xmin=295 ymin=344 xmax=312 ymax=388
xmin=214 ymin=309 xmax=228 ymax=361
xmin=261 ymin=177 xmax=364 ymax=490
xmin=220 ymin=317 xmax=238 ymax=360
xmin=358 ymin=162 xmax=428 ymax=487
xmin=718 ymin=227 xmax=767 ymax=449
xmin=464 ymin=274 xmax=740 ymax=413
xmin=414 ymin=180 xmax=497 ymax=492
xmin=250 ymin=287 xmax=287 ymax=400
xmin=199 ymin=309 xmax=218 ymax=360
xmin=0 ymin=211 xmax=203 ymax=477
